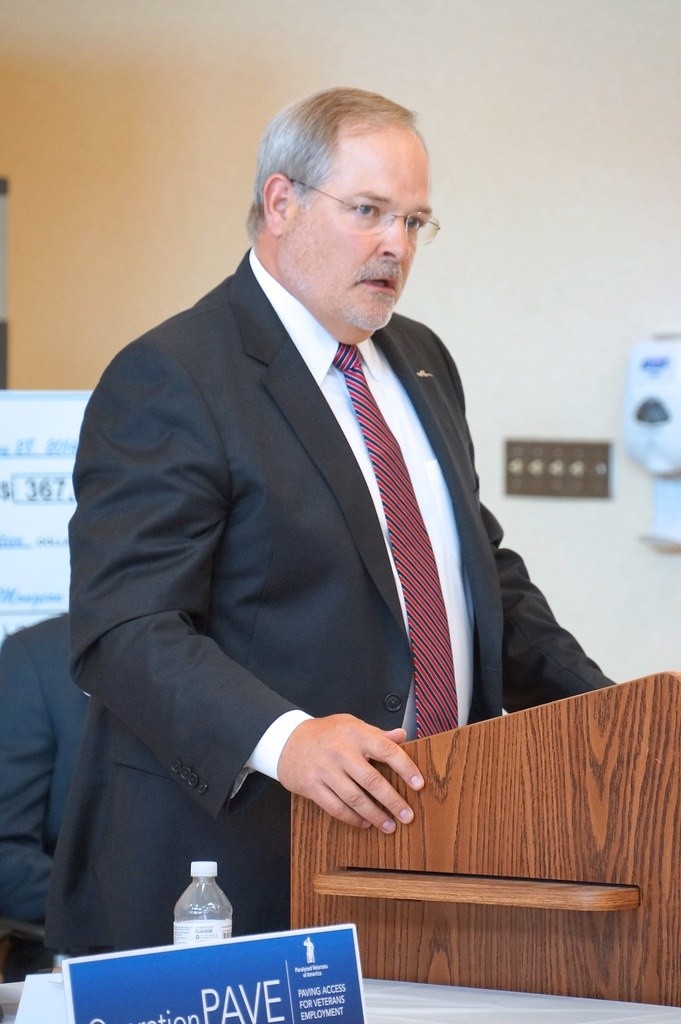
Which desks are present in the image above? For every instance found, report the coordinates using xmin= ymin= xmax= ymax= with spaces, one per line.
xmin=0 ymin=977 xmax=681 ymax=1024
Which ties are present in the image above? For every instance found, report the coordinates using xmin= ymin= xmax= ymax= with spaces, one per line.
xmin=332 ymin=342 xmax=458 ymax=741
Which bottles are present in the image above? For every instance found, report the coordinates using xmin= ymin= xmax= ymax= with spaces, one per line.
xmin=52 ymin=954 xmax=70 ymax=973
xmin=174 ymin=861 xmax=232 ymax=948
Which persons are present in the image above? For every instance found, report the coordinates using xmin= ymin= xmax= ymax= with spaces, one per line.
xmin=42 ymin=90 xmax=619 ymax=972
xmin=0 ymin=605 xmax=292 ymax=984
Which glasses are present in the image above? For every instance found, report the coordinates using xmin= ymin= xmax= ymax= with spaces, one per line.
xmin=288 ymin=179 xmax=441 ymax=245
